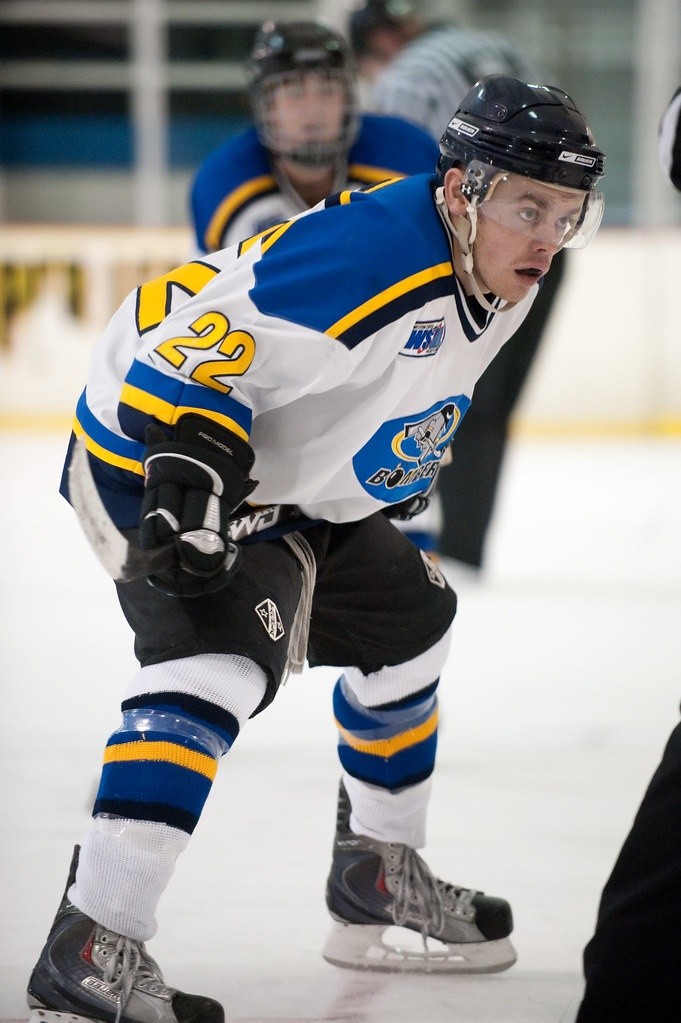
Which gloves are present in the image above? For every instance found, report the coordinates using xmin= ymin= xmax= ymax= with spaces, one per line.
xmin=138 ymin=409 xmax=260 ymax=594
xmin=386 ymin=496 xmax=428 ymax=526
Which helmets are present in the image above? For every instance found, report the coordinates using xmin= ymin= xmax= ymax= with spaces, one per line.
xmin=443 ymin=73 xmax=606 ymax=197
xmin=250 ymin=18 xmax=361 ymax=156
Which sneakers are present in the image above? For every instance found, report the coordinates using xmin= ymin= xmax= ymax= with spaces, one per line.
xmin=25 ymin=842 xmax=225 ymax=1022
xmin=320 ymin=780 xmax=517 ymax=975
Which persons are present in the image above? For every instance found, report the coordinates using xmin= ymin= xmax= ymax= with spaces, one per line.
xmin=346 ymin=0 xmax=566 ymax=576
xmin=25 ymin=76 xmax=605 ymax=1023
xmin=189 ymin=24 xmax=441 ymax=255
xmin=576 ymin=89 xmax=681 ymax=1023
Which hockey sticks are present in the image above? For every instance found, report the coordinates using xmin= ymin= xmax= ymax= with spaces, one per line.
xmin=65 ymin=436 xmax=304 ymax=584
